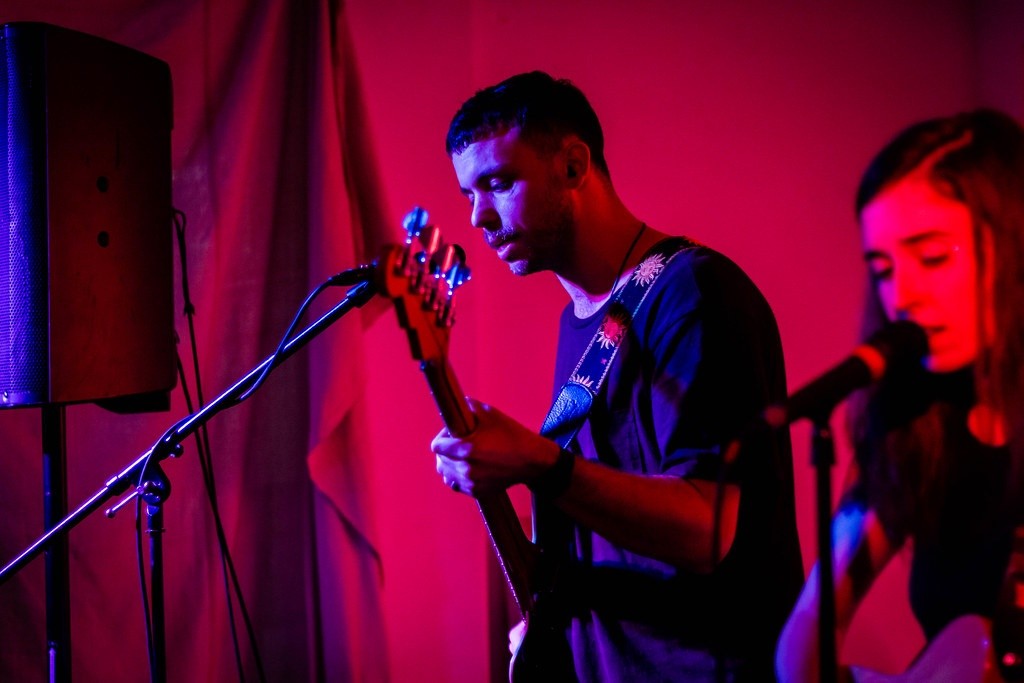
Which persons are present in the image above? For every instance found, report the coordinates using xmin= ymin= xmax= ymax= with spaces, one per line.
xmin=432 ymin=69 xmax=806 ymax=683
xmin=777 ymin=108 xmax=1024 ymax=683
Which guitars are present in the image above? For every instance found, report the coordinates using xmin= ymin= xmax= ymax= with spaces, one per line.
xmin=370 ymin=203 xmax=553 ymax=683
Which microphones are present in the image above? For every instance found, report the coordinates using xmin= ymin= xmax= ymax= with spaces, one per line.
xmin=327 ymin=243 xmax=466 ymax=290
xmin=762 ymin=320 xmax=930 ymax=433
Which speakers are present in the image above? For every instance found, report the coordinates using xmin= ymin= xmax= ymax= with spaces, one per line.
xmin=0 ymin=21 xmax=178 ymax=413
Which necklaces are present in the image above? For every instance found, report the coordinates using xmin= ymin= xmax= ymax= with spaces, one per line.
xmin=611 ymin=222 xmax=646 ymax=295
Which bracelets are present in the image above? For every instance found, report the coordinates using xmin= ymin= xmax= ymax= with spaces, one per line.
xmin=539 ymin=448 xmax=574 ymax=498
